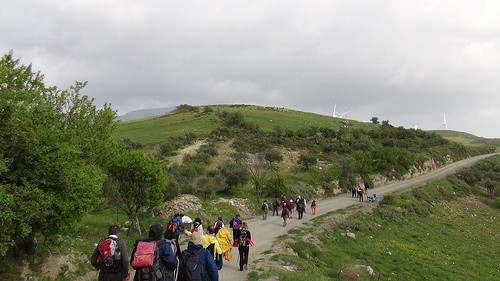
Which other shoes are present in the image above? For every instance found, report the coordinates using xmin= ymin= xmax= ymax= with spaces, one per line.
xmin=239 ymin=265 xmax=247 ymax=272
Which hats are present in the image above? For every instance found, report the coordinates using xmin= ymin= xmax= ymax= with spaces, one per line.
xmin=188 ymin=233 xmax=202 ymax=243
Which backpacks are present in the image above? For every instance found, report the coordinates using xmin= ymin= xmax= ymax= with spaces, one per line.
xmin=232 ymin=218 xmax=240 ymax=230
xmin=130 ymin=237 xmax=166 ymax=281
xmin=167 ymin=218 xmax=179 ymax=234
xmin=192 ymin=223 xmax=201 ymax=233
xmin=240 ymin=230 xmax=249 ymax=248
xmin=98 ymin=238 xmax=121 ymax=274
xmin=182 ymin=248 xmax=213 ymax=281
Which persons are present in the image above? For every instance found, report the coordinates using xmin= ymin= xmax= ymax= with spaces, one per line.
xmin=272 ymin=198 xmax=279 ymax=216
xmin=232 ymin=215 xmax=242 ymax=246
xmin=281 ymin=195 xmax=307 ymax=220
xmin=178 ymin=231 xmax=219 ymax=281
xmin=281 ymin=204 xmax=289 ymax=226
xmin=350 ymin=181 xmax=378 ymax=202
xmin=131 ymin=223 xmax=178 ymax=280
xmin=165 ymin=213 xmax=181 ymax=259
xmin=237 ymin=221 xmax=251 ymax=271
xmin=262 ymin=199 xmax=268 ymax=220
xmin=229 ymin=217 xmax=235 ymax=237
xmin=214 ymin=217 xmax=224 ymax=228
xmin=310 ymin=199 xmax=317 ymax=214
xmin=201 ymin=227 xmax=222 ymax=260
xmin=190 ymin=217 xmax=203 ymax=234
xmin=214 ymin=220 xmax=233 ymax=269
xmin=180 ymin=213 xmax=193 ymax=229
xmin=91 ymin=224 xmax=130 ymax=280
